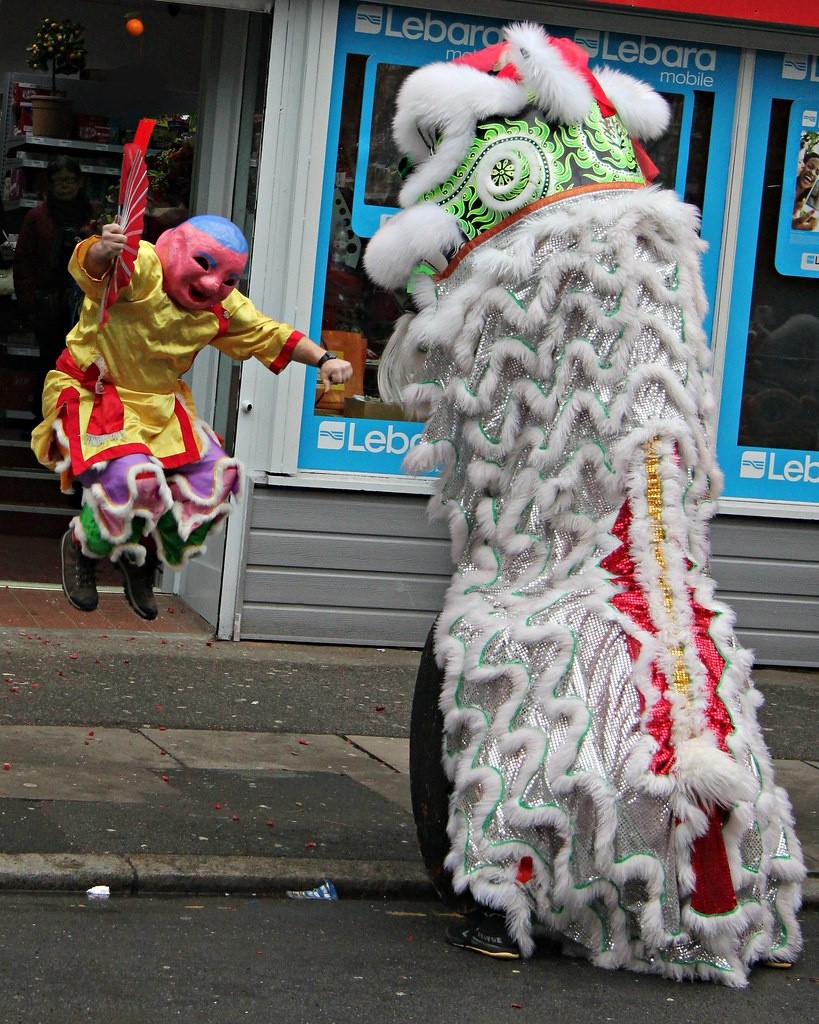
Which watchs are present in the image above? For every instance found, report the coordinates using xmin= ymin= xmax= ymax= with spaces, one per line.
xmin=317 ymin=351 xmax=337 ymax=370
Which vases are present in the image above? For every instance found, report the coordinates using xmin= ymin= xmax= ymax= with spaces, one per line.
xmin=31 ymin=95 xmax=74 ymax=139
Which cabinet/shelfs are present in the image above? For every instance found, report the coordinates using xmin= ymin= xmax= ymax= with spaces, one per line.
xmin=1 ymin=72 xmax=168 ymax=420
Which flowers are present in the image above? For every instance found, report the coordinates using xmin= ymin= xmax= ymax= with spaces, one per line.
xmin=25 ymin=16 xmax=86 ymax=95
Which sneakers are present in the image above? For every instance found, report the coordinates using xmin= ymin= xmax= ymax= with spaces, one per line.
xmin=445 ymin=925 xmax=521 ymax=958
xmin=120 ymin=558 xmax=159 ymax=620
xmin=61 ymin=526 xmax=100 ymax=613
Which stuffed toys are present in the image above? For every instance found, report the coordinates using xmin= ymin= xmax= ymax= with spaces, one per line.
xmin=360 ymin=16 xmax=810 ymax=989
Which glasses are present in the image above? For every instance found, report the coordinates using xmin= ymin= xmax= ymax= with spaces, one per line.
xmin=51 ymin=175 xmax=81 ymax=185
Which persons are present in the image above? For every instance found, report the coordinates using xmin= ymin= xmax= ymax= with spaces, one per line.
xmin=29 ymin=214 xmax=355 ymax=621
xmin=11 ymin=154 xmax=111 ymax=442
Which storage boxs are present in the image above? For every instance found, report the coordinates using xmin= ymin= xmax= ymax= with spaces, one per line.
xmin=12 ymin=80 xmax=112 ymax=143
xmin=343 ymin=397 xmax=401 ymax=420
